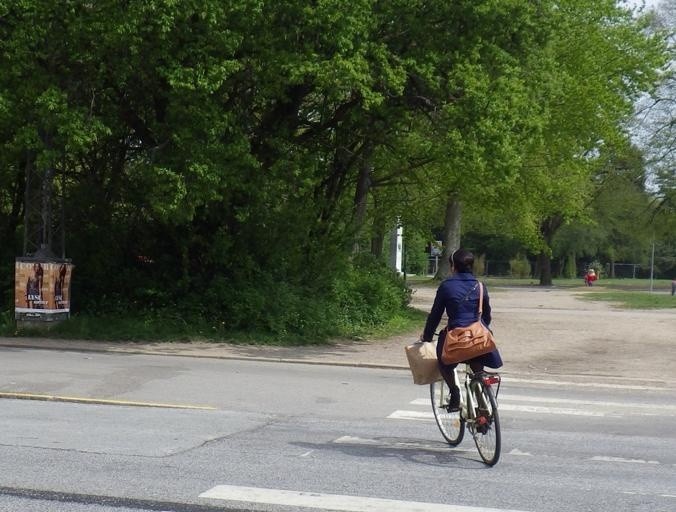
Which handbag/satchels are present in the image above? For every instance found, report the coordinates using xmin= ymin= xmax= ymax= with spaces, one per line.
xmin=404 ymin=336 xmax=446 ymax=386
xmin=440 ymin=320 xmax=499 ymax=367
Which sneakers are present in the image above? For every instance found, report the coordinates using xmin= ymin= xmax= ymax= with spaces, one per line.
xmin=448 ymin=386 xmax=461 ymax=409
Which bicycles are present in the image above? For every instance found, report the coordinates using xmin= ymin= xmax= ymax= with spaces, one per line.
xmin=422 ymin=327 xmax=505 ymax=466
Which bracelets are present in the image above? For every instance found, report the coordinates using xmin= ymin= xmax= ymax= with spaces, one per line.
xmin=24 ymin=295 xmax=27 ymax=296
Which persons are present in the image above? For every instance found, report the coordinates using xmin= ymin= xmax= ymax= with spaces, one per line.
xmin=585 ymin=272 xmax=588 ymax=287
xmin=671 ymin=278 xmax=676 ymax=295
xmin=418 ymin=246 xmax=504 ymax=434
xmin=587 ymin=271 xmax=594 ymax=287
xmin=22 ymin=263 xmax=44 ymax=310
xmin=51 ymin=264 xmax=67 ymax=310
